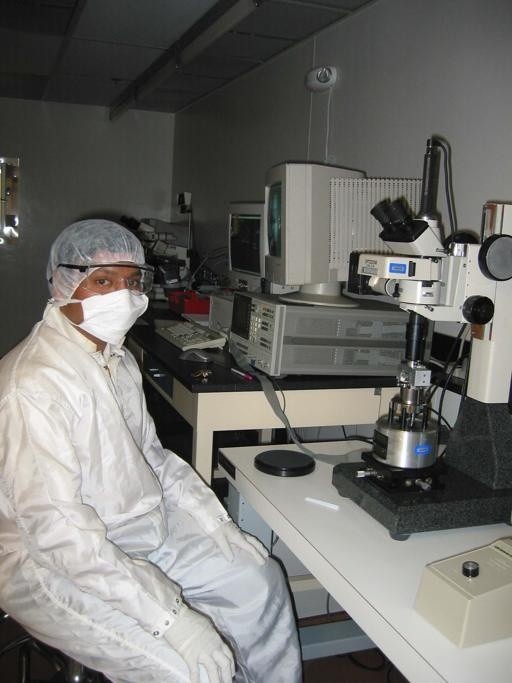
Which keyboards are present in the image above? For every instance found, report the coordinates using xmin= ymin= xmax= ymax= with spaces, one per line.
xmin=155 ymin=320 xmax=226 ymax=352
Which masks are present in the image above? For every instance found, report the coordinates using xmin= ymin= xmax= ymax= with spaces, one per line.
xmin=78 ymin=288 xmax=149 ymax=345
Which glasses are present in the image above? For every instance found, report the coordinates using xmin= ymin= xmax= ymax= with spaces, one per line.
xmin=49 ymin=264 xmax=155 ymax=296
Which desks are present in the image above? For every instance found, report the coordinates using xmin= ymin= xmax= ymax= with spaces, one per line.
xmin=216 ymin=437 xmax=511 ymax=683
xmin=125 ymin=306 xmax=463 ymax=491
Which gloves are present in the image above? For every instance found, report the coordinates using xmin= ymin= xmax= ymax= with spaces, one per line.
xmin=211 ymin=520 xmax=269 ymax=566
xmin=163 ymin=606 xmax=236 ymax=683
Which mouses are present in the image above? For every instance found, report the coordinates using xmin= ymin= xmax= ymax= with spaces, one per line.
xmin=178 ymin=348 xmax=213 ymax=363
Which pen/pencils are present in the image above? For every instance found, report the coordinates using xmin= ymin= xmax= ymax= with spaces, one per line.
xmin=230 ymin=368 xmax=252 ymax=381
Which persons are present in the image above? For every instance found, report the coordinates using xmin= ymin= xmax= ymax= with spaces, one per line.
xmin=0 ymin=217 xmax=306 ymax=683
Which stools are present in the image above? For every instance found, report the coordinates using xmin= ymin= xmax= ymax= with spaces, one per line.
xmin=0 ymin=608 xmax=111 ymax=683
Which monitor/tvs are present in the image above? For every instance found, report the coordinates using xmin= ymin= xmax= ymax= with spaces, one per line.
xmin=227 ymin=202 xmax=265 ymax=291
xmin=265 ymin=163 xmax=366 ymax=308
